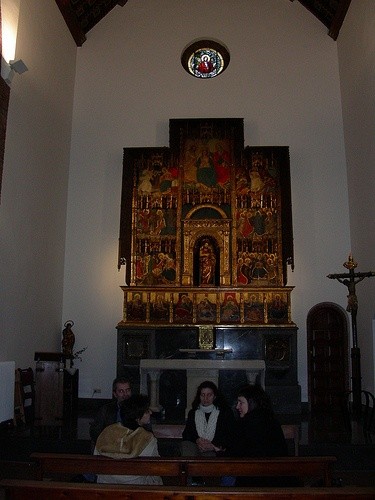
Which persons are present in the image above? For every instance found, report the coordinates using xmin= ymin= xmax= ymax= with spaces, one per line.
xmin=62 ymin=323 xmax=75 ymax=355
xmin=89 ymin=377 xmax=133 ymax=452
xmin=209 ymin=384 xmax=298 ymax=487
xmin=200 ymin=239 xmax=212 ymax=284
xmin=93 ymin=394 xmax=165 ymax=485
xmin=179 ymin=381 xmax=236 ymax=486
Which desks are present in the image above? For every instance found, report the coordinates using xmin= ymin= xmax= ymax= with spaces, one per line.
xmin=139 ymin=358 xmax=266 ymax=421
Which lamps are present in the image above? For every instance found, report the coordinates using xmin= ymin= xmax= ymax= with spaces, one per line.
xmin=181 ymin=37 xmax=230 ymax=79
xmin=3 ymin=58 xmax=29 ymax=85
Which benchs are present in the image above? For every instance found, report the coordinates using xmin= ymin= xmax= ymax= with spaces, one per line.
xmin=0 ymin=453 xmax=375 ymax=500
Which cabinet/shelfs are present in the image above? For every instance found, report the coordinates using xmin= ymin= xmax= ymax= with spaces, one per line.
xmin=33 ymin=351 xmax=79 ymax=441
